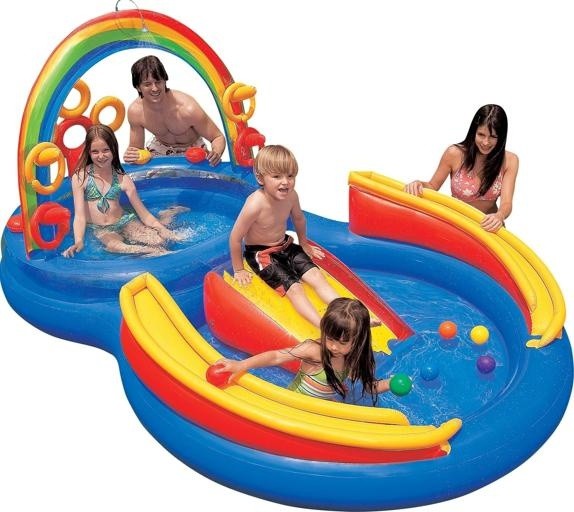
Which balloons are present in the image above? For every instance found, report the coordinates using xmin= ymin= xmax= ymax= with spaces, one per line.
xmin=389 ymin=373 xmax=413 ymax=396
xmin=467 ymin=324 xmax=489 ymax=344
xmin=420 ymin=362 xmax=437 ymax=382
xmin=438 ymin=319 xmax=458 ymax=341
xmin=206 ymin=364 xmax=229 ymax=388
xmin=185 ymin=146 xmax=205 ymax=164
xmin=477 ymin=354 xmax=494 ymax=375
xmin=134 ymin=148 xmax=150 ymax=164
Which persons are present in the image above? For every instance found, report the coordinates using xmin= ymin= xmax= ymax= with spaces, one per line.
xmin=227 ymin=144 xmax=382 ymax=330
xmin=123 ymin=56 xmax=226 ymax=167
xmin=404 ymin=105 xmax=519 ymax=235
xmin=213 ymin=296 xmax=415 ymax=405
xmin=61 ymin=124 xmax=192 ymax=261
